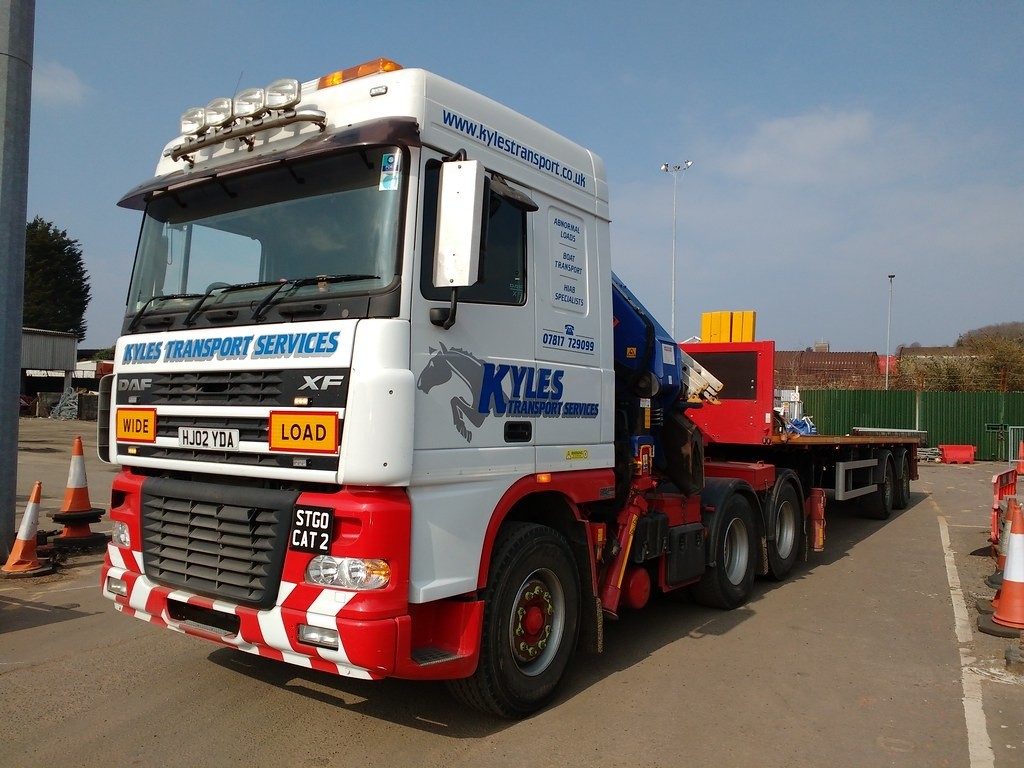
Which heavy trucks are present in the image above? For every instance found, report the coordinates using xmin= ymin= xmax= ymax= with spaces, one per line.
xmin=95 ymin=58 xmax=921 ymax=725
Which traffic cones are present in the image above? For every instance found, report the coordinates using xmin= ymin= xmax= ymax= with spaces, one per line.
xmin=51 ymin=435 xmax=106 ymax=526
xmin=0 ymin=480 xmax=55 ymax=579
xmin=975 ymin=588 xmax=1000 ymax=615
xmin=984 ymin=497 xmax=1018 ymax=590
xmin=978 ymin=504 xmax=1024 ymax=639
xmin=1015 ymin=440 xmax=1024 ymax=475
xmin=46 ymin=521 xmax=106 ymax=547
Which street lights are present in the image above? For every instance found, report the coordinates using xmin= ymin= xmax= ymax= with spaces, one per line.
xmin=885 ymin=273 xmax=896 ymax=391
xmin=661 ymin=158 xmax=694 ymax=341
